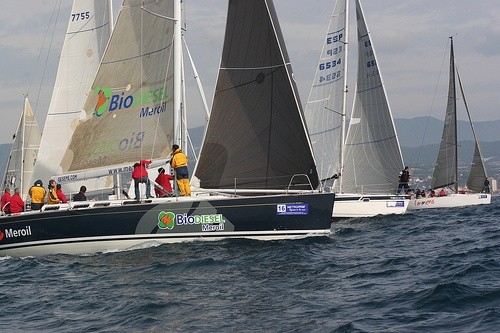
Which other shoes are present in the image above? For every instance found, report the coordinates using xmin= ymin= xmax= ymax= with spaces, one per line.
xmin=134 ymin=193 xmax=192 ymax=200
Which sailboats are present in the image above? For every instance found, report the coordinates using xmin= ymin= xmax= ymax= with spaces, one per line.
xmin=407 ymin=34 xmax=491 ymax=209
xmin=0 ymin=0 xmax=335 ymax=257
xmin=304 ymin=0 xmax=412 ymax=218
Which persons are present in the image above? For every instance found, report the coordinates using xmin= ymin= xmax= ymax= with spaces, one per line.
xmin=155 ymin=168 xmax=175 ymax=197
xmin=48 ymin=180 xmax=60 ymax=210
xmin=395 ymin=166 xmax=410 ymax=195
xmin=74 ymin=186 xmax=89 ymax=208
xmin=170 ymin=144 xmax=191 ymax=196
xmin=9 ymin=188 xmax=25 ymax=213
xmin=28 ymin=179 xmax=46 ymax=210
xmin=132 ymin=160 xmax=154 ymax=200
xmin=1 ymin=187 xmax=12 ymax=214
xmin=405 ymin=189 xmax=446 ymax=199
xmin=113 ymin=185 xmax=131 ymax=199
xmin=56 ymin=184 xmax=67 ymax=203
xmin=482 ymin=178 xmax=490 ymax=193
